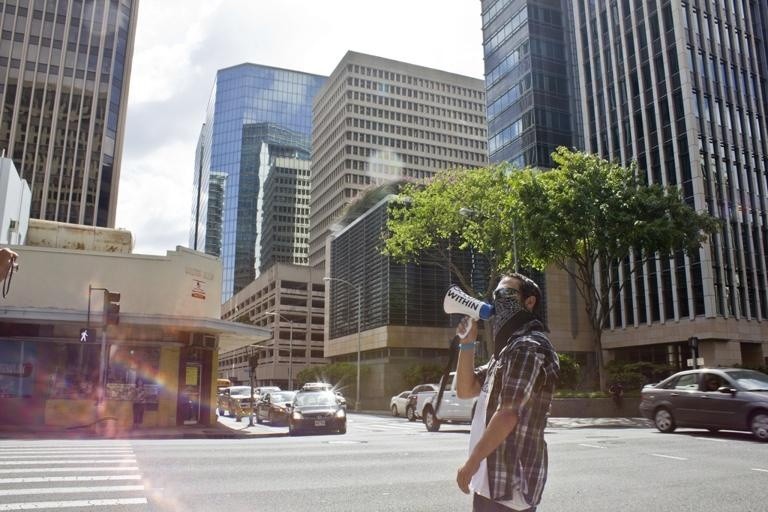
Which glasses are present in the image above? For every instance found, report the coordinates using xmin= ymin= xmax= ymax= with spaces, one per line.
xmin=492 ymin=287 xmax=520 ymax=300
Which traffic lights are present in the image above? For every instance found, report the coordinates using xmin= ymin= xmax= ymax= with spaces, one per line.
xmin=689 ymin=336 xmax=698 ymax=348
xmin=80 ymin=328 xmax=95 ymax=341
xmin=103 ymin=290 xmax=121 ymax=326
xmin=250 ymin=356 xmax=258 ymax=368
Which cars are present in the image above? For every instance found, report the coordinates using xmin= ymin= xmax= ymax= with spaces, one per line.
xmin=638 ymin=366 xmax=768 ymax=441
xmin=216 ymin=378 xmax=347 ymax=434
xmin=404 ymin=383 xmax=439 ymax=421
xmin=390 ymin=389 xmax=412 ymax=416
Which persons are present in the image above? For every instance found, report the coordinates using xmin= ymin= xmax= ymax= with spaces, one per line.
xmin=454 ymin=273 xmax=560 ymax=511
xmin=706 ymin=378 xmax=719 ymax=391
xmin=129 ymin=379 xmax=146 ymax=429
xmin=608 ymin=382 xmax=624 ymax=408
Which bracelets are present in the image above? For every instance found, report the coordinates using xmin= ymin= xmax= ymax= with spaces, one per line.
xmin=459 ymin=343 xmax=477 ymax=350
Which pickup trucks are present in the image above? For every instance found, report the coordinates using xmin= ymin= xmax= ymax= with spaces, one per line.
xmin=414 ymin=370 xmax=481 ymax=432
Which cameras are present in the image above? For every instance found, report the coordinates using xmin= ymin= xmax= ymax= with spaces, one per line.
xmin=7 ymin=255 xmax=19 ymax=273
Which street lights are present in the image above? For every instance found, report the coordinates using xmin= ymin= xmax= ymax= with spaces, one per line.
xmin=323 ymin=277 xmax=361 ymax=414
xmin=453 ymin=205 xmax=517 ymax=276
xmin=265 ymin=312 xmax=294 ymax=389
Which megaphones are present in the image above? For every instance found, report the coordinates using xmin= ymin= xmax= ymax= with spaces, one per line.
xmin=441 ymin=283 xmax=494 ymax=339
